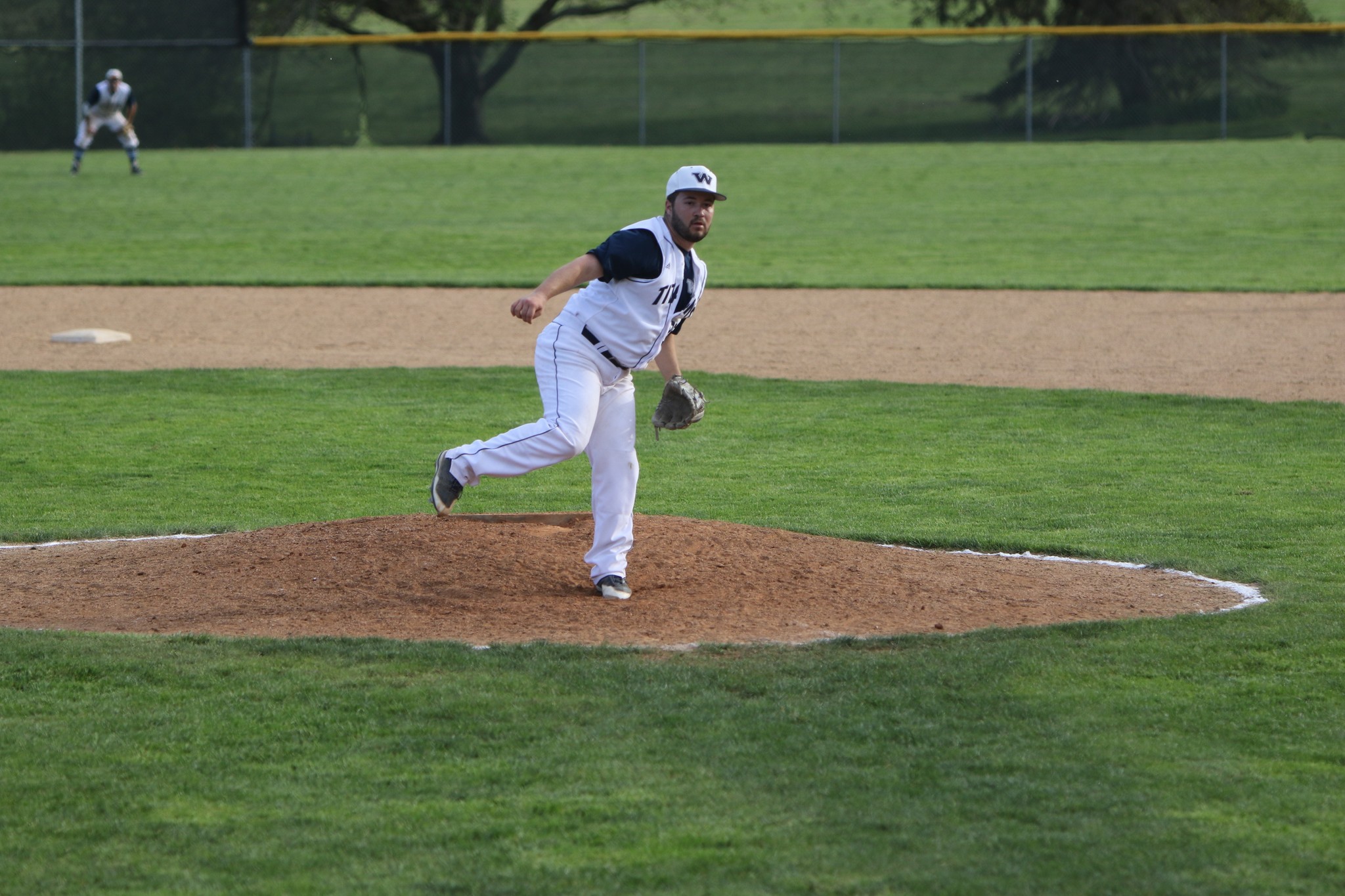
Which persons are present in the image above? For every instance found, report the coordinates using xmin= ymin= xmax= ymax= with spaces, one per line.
xmin=432 ymin=165 xmax=729 ymax=599
xmin=68 ymin=70 xmax=143 ymax=176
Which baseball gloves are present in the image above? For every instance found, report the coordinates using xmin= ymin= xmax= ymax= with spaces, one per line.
xmin=652 ymin=374 xmax=705 ymax=430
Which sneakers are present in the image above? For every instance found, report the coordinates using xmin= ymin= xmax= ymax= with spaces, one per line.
xmin=429 ymin=450 xmax=463 ymax=516
xmin=595 ymin=574 xmax=632 ymax=601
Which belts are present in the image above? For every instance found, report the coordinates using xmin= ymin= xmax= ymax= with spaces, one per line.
xmin=582 ymin=328 xmax=630 ymax=371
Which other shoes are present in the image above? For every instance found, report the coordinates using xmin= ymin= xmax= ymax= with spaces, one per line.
xmin=70 ymin=166 xmax=79 ymax=173
xmin=131 ymin=167 xmax=140 ymax=173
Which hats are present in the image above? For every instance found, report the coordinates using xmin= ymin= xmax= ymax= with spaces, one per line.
xmin=107 ymin=68 xmax=122 ymax=80
xmin=666 ymin=166 xmax=726 ymax=201
